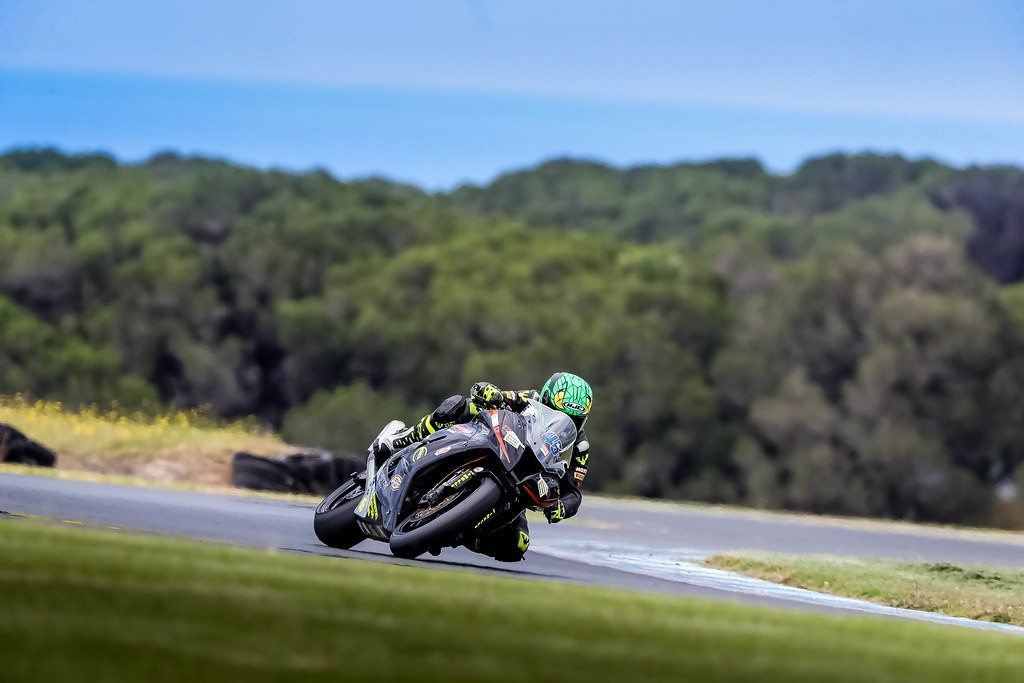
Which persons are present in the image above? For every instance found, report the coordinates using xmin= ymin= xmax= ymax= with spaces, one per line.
xmin=379 ymin=371 xmax=592 ymax=562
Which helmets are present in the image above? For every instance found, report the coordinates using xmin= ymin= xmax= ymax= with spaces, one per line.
xmin=539 ymin=371 xmax=593 ymax=436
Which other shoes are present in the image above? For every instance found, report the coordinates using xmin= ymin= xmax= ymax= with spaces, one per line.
xmin=373 ymin=419 xmax=418 ymax=474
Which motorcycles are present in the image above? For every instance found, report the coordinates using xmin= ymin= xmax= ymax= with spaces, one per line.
xmin=314 ymin=397 xmax=578 ymax=559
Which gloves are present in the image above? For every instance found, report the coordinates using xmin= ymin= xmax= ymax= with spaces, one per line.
xmin=471 ymin=383 xmax=504 ymax=410
xmin=544 ymin=490 xmax=583 ymax=524
